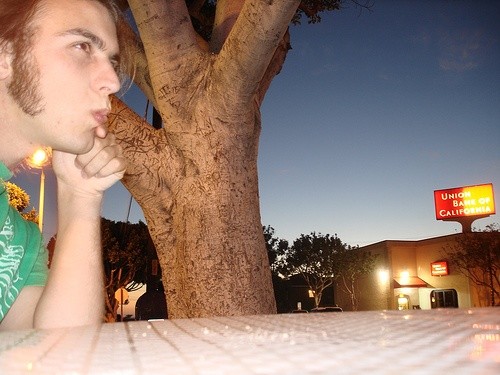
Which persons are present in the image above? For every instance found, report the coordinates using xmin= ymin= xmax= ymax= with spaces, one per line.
xmin=1 ymin=0 xmax=131 ymax=331
